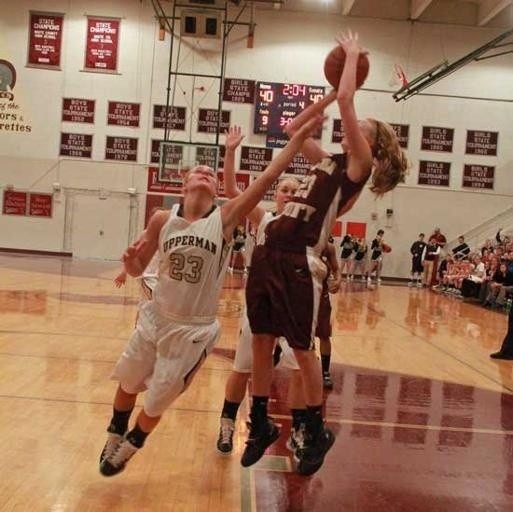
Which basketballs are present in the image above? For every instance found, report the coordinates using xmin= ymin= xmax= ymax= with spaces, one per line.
xmin=324 ymin=47 xmax=369 ymax=90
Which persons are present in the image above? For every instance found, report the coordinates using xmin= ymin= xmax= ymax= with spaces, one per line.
xmin=316 ymin=241 xmax=339 ymax=390
xmin=490 ymin=301 xmax=513 ymax=360
xmin=216 ymin=124 xmax=311 ymax=457
xmin=328 ymin=229 xmax=392 ymax=288
xmin=407 ymin=226 xmax=512 ymax=311
xmin=240 ymin=27 xmax=408 ymax=472
xmin=94 ymin=112 xmax=330 ymax=475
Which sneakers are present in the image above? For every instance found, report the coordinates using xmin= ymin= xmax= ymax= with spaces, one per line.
xmin=241 ymin=415 xmax=279 ymax=467
xmin=216 ymin=418 xmax=234 ymax=455
xmin=340 ymin=274 xmax=381 ymax=282
xmin=322 ymin=370 xmax=333 ymax=388
xmin=296 ymin=428 xmax=336 ymax=476
xmin=489 ymin=350 xmax=512 ymax=360
xmin=289 ymin=422 xmax=306 ymax=462
xmin=100 ymin=424 xmax=129 ymax=464
xmin=408 ymin=280 xmax=463 ymax=297
xmin=99 ymin=428 xmax=143 ymax=477
